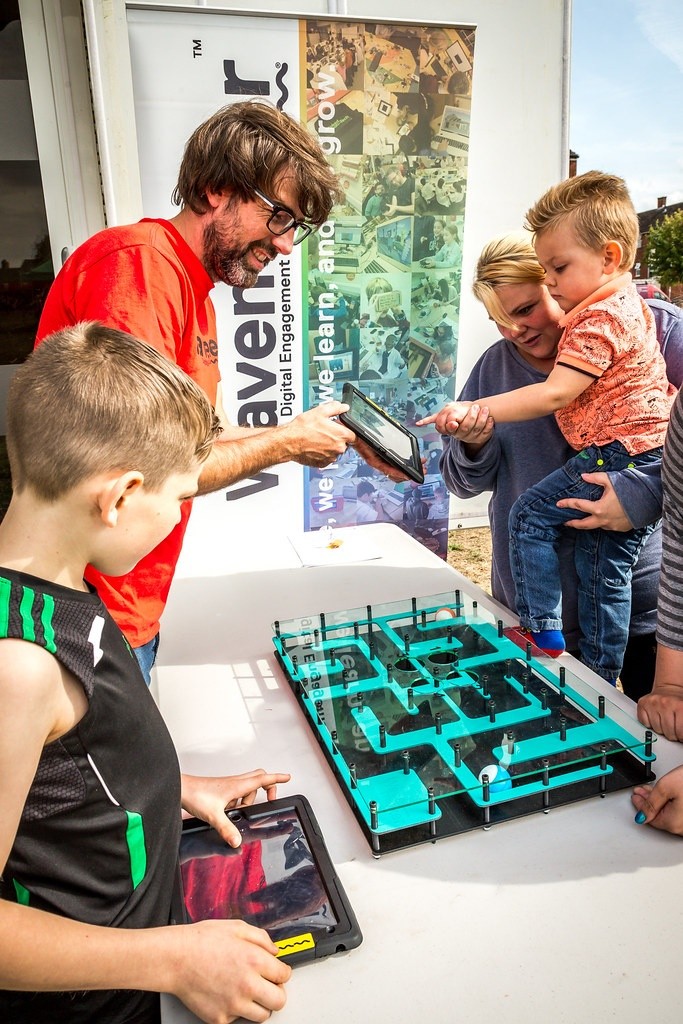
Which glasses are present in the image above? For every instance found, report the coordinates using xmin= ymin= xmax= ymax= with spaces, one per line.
xmin=254 ymin=189 xmax=311 ymax=246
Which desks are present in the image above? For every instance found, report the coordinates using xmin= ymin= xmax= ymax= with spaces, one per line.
xmin=148 ymin=523 xmax=683 ymax=1024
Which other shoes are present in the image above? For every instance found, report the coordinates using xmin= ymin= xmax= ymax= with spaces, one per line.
xmin=504 ymin=626 xmax=566 ymax=658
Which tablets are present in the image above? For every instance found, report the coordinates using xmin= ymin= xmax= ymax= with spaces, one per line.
xmin=170 ymin=794 xmax=363 ymax=965
xmin=338 ymin=383 xmax=423 ymax=485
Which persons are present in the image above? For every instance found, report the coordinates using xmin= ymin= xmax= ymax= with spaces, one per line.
xmin=0 ymin=322 xmax=292 ymax=1024
xmin=0 ymin=97 xmax=356 ymax=696
xmin=415 ymin=170 xmax=683 ymax=838
